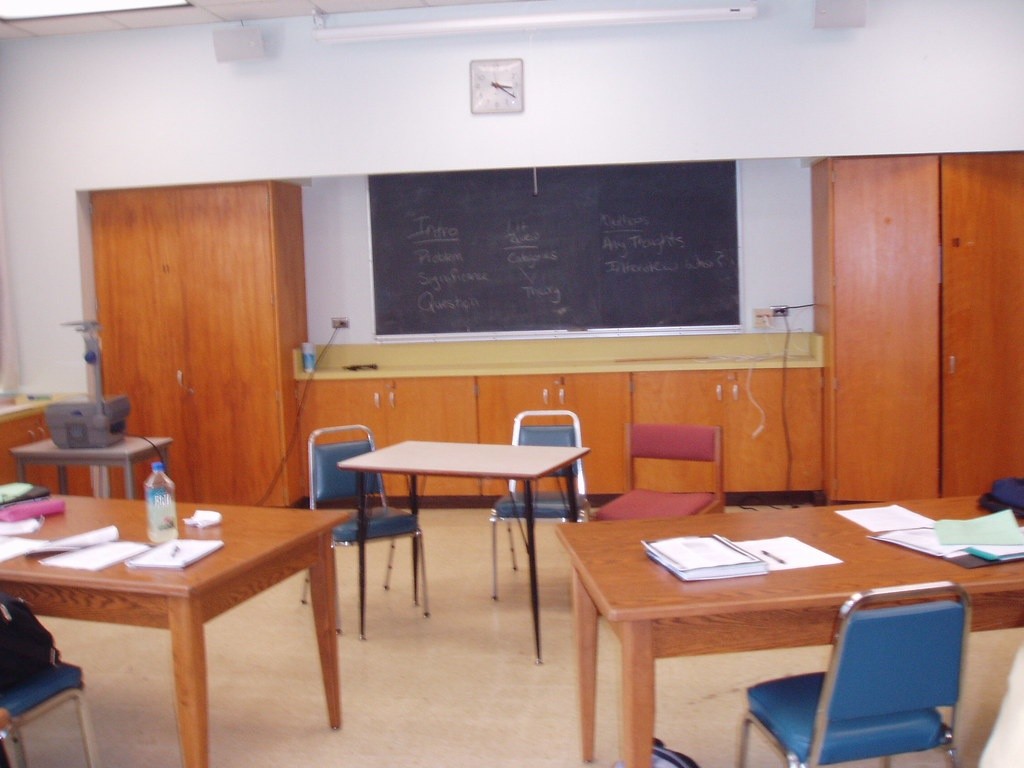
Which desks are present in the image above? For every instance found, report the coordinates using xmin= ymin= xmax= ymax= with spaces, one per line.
xmin=338 ymin=440 xmax=593 ymax=666
xmin=9 ymin=436 xmax=173 ymax=500
xmin=553 ymin=494 xmax=1024 ymax=768
xmin=0 ymin=495 xmax=356 ymax=768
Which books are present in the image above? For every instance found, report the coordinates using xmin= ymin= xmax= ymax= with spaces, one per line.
xmin=126 ymin=539 xmax=224 ymax=567
xmin=642 ymin=537 xmax=769 ymax=581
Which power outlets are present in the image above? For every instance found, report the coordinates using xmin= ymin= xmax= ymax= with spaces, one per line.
xmin=754 ymin=308 xmax=775 ymax=328
xmin=331 ymin=318 xmax=348 ymax=328
xmin=770 ymin=306 xmax=788 ymax=317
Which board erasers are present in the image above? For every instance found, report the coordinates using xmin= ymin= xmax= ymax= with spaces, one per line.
xmin=566 ymin=325 xmax=588 ymax=332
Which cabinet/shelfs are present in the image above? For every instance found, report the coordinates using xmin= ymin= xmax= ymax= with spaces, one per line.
xmin=291 ymin=368 xmax=826 ymax=509
xmin=92 ymin=180 xmax=308 ymax=509
xmin=812 ymin=150 xmax=1024 ymax=505
xmin=0 ymin=412 xmax=92 ymax=495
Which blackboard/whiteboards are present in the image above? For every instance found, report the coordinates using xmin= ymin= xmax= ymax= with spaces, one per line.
xmin=366 ymin=158 xmax=753 ymax=342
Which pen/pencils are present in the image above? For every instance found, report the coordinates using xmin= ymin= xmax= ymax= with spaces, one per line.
xmin=760 ymin=547 xmax=787 ymax=570
xmin=170 ymin=543 xmax=181 ymax=562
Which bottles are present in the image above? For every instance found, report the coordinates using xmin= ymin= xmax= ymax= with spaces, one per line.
xmin=144 ymin=462 xmax=179 ymax=543
xmin=302 ymin=342 xmax=315 ymax=373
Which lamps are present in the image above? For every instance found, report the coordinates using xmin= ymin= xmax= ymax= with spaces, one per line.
xmin=308 ymin=0 xmax=759 ymax=43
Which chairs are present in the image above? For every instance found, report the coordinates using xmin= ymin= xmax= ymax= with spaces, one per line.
xmin=0 ymin=595 xmax=102 ymax=768
xmin=489 ymin=411 xmax=588 ymax=603
xmin=302 ymin=424 xmax=432 ymax=639
xmin=588 ymin=420 xmax=726 ymax=521
xmin=736 ymin=579 xmax=972 ymax=768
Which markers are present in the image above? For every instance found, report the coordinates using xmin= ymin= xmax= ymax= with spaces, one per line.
xmin=344 ymin=363 xmax=378 ymax=371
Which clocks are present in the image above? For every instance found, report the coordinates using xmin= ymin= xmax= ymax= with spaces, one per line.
xmin=469 ymin=58 xmax=523 ymax=114
